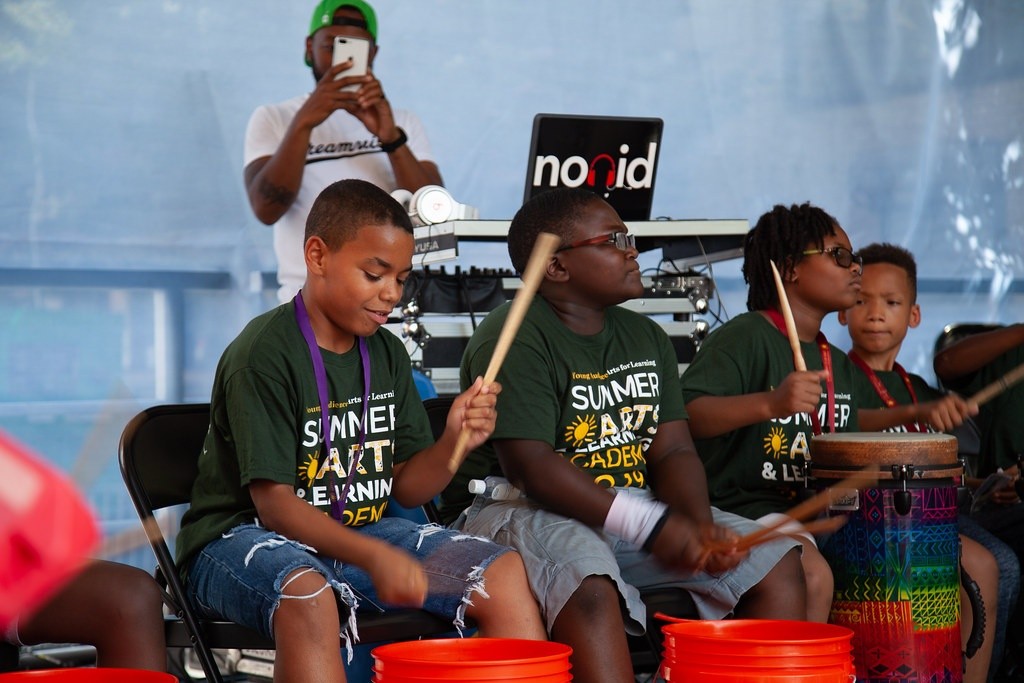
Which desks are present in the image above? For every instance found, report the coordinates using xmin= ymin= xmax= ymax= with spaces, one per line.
xmin=382 ymin=218 xmax=751 ymax=396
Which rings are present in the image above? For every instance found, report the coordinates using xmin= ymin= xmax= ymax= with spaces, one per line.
xmin=381 ymin=95 xmax=384 ymax=98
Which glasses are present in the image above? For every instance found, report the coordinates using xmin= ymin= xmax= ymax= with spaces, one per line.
xmin=556 ymin=231 xmax=636 ymax=254
xmin=801 ymin=248 xmax=863 ymax=276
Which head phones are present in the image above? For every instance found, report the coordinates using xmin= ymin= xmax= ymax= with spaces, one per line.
xmin=391 ymin=184 xmax=480 ymax=227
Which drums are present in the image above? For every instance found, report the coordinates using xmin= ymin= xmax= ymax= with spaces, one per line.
xmin=810 ymin=430 xmax=965 ymax=682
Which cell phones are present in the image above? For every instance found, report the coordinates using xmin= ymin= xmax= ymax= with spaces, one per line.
xmin=330 ymin=35 xmax=371 ymax=92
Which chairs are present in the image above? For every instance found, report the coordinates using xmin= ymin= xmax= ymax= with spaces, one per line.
xmin=925 ymin=322 xmax=1024 ymax=561
xmin=0 ymin=392 xmax=508 ymax=683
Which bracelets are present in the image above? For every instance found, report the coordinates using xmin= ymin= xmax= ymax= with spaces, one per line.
xmin=381 ymin=127 xmax=407 ymax=153
xmin=603 ymin=491 xmax=670 ymax=552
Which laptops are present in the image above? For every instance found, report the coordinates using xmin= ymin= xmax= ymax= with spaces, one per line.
xmin=524 ymin=113 xmax=664 ymax=221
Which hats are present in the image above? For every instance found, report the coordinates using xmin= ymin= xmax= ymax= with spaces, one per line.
xmin=305 ymin=0 xmax=377 ymax=68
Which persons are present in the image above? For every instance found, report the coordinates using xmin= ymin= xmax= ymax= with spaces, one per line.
xmin=837 ymin=242 xmax=1024 ymax=683
xmin=677 ymin=201 xmax=997 ymax=683
xmin=242 ymin=1 xmax=445 ymax=308
xmin=0 ymin=557 xmax=167 ymax=673
xmin=177 ymin=178 xmax=548 ymax=682
xmin=459 ymin=187 xmax=809 ymax=681
